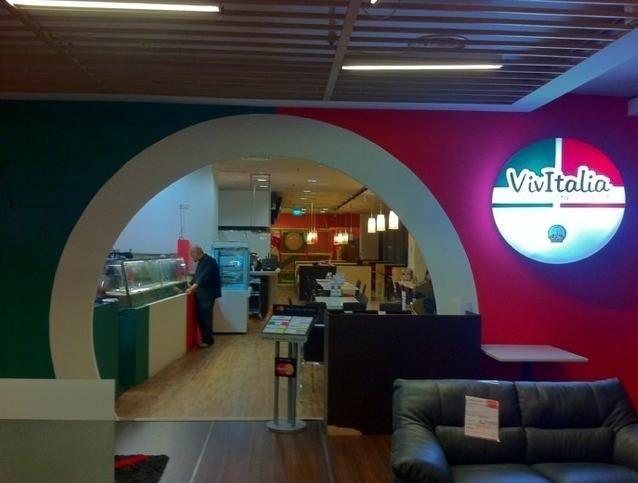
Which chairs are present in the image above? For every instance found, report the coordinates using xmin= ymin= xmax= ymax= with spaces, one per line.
xmin=299 ymin=267 xmax=411 ymax=314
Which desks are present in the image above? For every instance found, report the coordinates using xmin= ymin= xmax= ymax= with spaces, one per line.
xmin=483 ymin=343 xmax=587 ymax=380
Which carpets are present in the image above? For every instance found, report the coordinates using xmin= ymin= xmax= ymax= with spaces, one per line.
xmin=116 ymin=454 xmax=167 ymax=482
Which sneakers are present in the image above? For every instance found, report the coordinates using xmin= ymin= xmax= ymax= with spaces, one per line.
xmin=198 ymin=343 xmax=209 ymax=347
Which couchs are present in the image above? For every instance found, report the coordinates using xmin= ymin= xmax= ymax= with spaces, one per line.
xmin=390 ymin=375 xmax=638 ymax=481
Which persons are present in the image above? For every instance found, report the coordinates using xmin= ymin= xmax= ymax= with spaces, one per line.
xmin=185 ymin=244 xmax=222 ymax=348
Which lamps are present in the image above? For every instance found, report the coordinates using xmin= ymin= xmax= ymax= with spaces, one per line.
xmin=366 ymin=189 xmax=399 ymax=233
